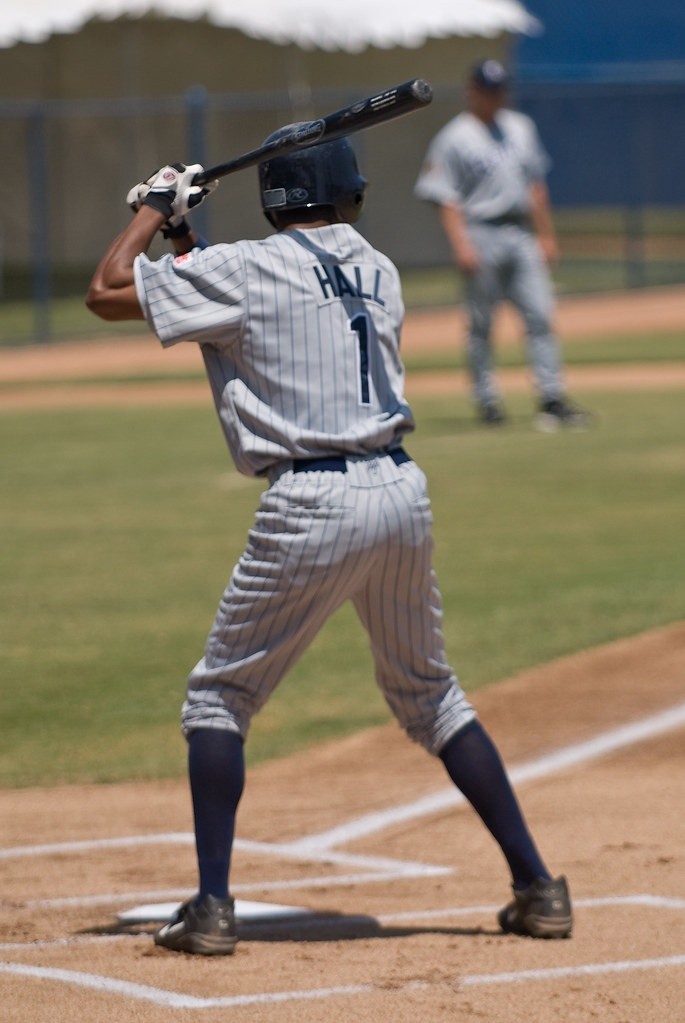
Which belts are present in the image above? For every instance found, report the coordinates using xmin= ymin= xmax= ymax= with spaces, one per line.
xmin=293 ymin=446 xmax=413 ymax=472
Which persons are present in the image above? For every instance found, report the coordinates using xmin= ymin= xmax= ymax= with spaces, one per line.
xmin=410 ymin=59 xmax=599 ymax=425
xmin=82 ymin=119 xmax=574 ymax=954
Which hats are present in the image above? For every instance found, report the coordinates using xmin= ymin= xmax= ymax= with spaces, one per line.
xmin=464 ymin=59 xmax=511 ymax=88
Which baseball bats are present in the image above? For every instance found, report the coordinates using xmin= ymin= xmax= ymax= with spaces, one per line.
xmin=193 ymin=77 xmax=436 ymax=187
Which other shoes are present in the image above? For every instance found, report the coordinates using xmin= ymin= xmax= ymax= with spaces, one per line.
xmin=478 ymin=402 xmax=504 ymax=424
xmin=539 ymin=396 xmax=589 ymax=422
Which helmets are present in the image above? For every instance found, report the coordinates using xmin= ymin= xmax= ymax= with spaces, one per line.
xmin=258 ymin=121 xmax=369 ymax=226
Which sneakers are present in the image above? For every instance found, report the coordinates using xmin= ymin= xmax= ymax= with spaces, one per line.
xmin=497 ymin=875 xmax=572 ymax=936
xmin=154 ymin=892 xmax=238 ymax=955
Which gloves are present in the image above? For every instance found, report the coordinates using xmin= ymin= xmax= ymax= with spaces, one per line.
xmin=126 ymin=163 xmax=220 ymax=237
